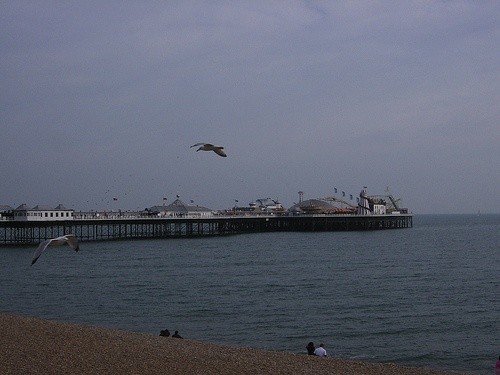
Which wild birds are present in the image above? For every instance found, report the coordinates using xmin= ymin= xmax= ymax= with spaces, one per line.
xmin=31 ymin=234 xmax=80 ymax=265
xmin=190 ymin=143 xmax=227 ymax=157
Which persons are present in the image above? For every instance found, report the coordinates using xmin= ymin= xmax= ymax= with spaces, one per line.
xmin=172 ymin=331 xmax=184 ymax=339
xmin=159 ymin=329 xmax=170 ymax=337
xmin=306 ymin=342 xmax=327 ymax=357
